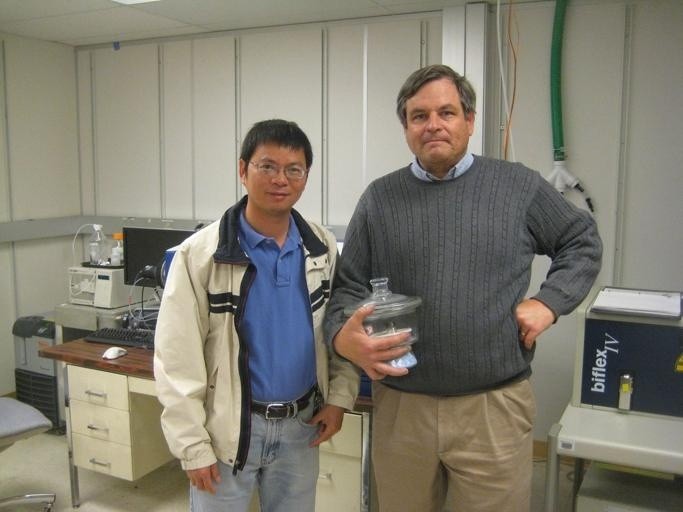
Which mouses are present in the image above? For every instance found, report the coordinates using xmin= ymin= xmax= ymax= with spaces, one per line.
xmin=102 ymin=346 xmax=127 ymax=360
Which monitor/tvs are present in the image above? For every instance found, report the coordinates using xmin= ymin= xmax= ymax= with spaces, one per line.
xmin=123 ymin=228 xmax=196 ymax=288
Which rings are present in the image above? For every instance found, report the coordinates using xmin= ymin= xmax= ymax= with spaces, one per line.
xmin=520 ymin=331 xmax=526 ymax=336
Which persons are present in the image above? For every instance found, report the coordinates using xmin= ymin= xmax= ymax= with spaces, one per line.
xmin=320 ymin=65 xmax=604 ymax=512
xmin=150 ymin=119 xmax=360 ymax=512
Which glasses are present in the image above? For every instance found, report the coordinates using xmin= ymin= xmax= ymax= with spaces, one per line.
xmin=246 ymin=161 xmax=308 ymax=179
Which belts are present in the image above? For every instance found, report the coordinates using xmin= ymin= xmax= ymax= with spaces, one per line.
xmin=252 ymin=382 xmax=317 ymax=419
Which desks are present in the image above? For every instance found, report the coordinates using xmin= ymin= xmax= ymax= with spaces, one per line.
xmin=38 ymin=336 xmax=373 ymax=512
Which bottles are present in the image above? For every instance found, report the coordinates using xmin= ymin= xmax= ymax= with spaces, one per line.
xmin=342 ymin=277 xmax=423 ymax=369
xmin=87 ymin=224 xmax=123 ymax=265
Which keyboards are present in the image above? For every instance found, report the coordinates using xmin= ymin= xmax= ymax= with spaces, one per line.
xmin=85 ymin=327 xmax=154 ymax=349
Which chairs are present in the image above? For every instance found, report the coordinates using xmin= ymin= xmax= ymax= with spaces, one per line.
xmin=0 ymin=396 xmax=57 ymax=512
